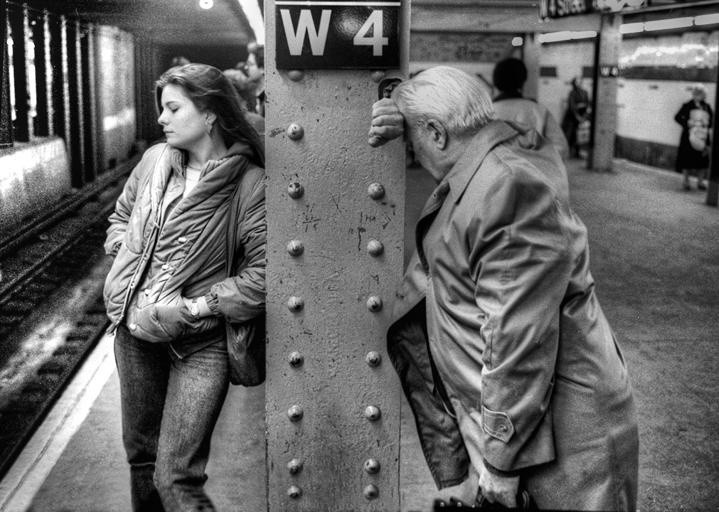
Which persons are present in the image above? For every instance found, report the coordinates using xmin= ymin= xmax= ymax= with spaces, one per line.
xmin=222 ymin=43 xmax=265 ymax=142
xmin=102 ymin=65 xmax=266 ymax=510
xmin=560 ymin=78 xmax=589 ymax=159
xmin=368 ymin=63 xmax=640 ymax=512
xmin=675 ymin=83 xmax=714 ymax=190
xmin=492 ymin=48 xmax=529 ymax=99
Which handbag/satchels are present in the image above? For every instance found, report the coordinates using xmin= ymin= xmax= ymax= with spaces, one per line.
xmin=224 ymin=308 xmax=263 ymax=387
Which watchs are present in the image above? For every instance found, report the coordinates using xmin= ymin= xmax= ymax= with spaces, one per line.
xmin=190 ymin=296 xmax=201 ymax=321
